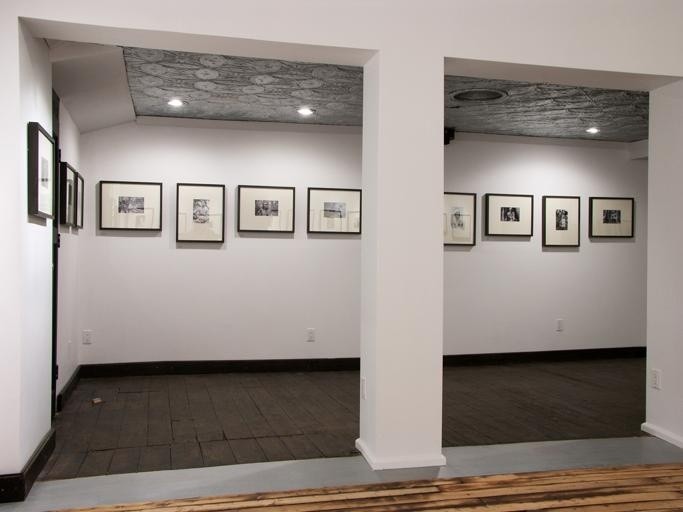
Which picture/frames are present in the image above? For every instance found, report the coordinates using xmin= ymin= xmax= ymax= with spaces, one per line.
xmin=485 ymin=193 xmax=534 ymax=237
xmin=175 ymin=183 xmax=226 ymax=243
xmin=237 ymin=184 xmax=295 ymax=232
xmin=307 ymin=187 xmax=362 ymax=235
xmin=443 ymin=192 xmax=477 ymax=247
xmin=542 ymin=195 xmax=581 ymax=247
xmin=99 ymin=180 xmax=163 ymax=232
xmin=27 ymin=121 xmax=86 ymax=231
xmin=588 ymin=196 xmax=635 ymax=239
